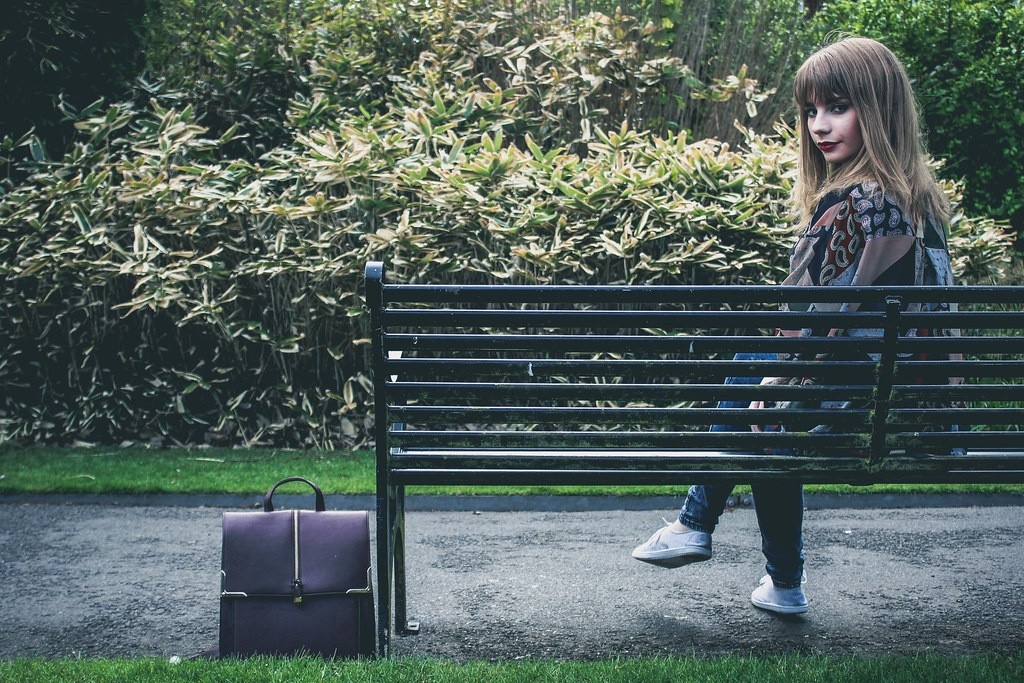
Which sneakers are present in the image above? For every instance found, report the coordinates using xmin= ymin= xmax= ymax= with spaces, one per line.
xmin=632 ymin=516 xmax=711 ymax=569
xmin=751 ymin=574 xmax=809 ymax=613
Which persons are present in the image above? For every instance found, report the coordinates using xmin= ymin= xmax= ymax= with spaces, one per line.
xmin=632 ymin=37 xmax=949 ymax=613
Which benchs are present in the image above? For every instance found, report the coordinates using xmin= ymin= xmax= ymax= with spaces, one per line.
xmin=363 ymin=260 xmax=1024 ymax=664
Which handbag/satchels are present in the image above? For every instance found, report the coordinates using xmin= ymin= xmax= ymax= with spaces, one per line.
xmin=219 ymin=476 xmax=376 ymax=660
